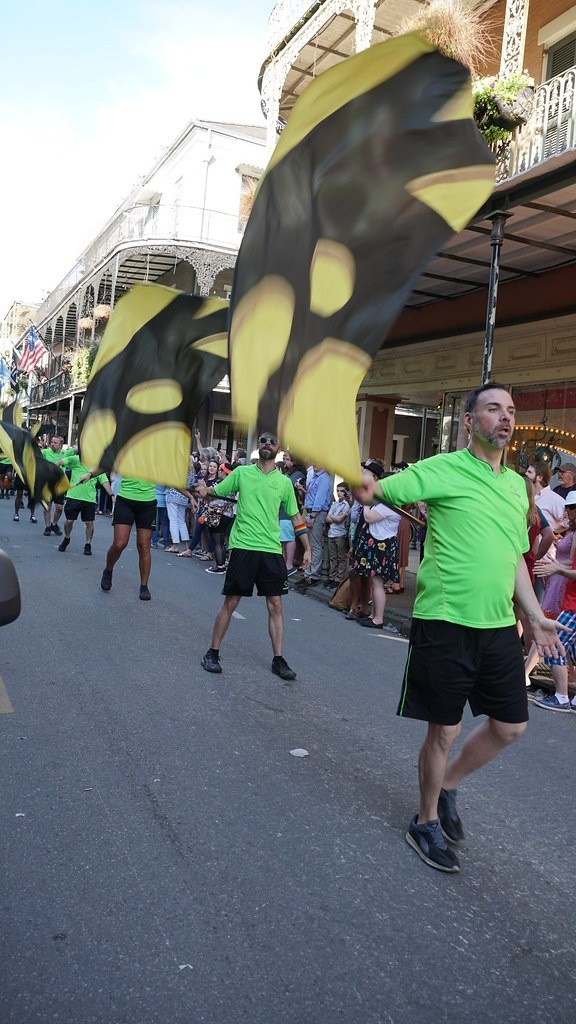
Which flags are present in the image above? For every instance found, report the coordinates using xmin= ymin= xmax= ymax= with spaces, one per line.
xmin=10 ymin=348 xmax=28 ymax=392
xmin=0 ymin=399 xmax=76 ymax=511
xmin=229 ymin=24 xmax=500 ymax=494
xmin=0 ymin=357 xmax=11 ymax=394
xmin=17 ymin=325 xmax=48 ymax=375
xmin=76 ymin=281 xmax=231 ymax=488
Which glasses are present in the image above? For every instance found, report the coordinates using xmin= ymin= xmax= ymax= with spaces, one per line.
xmin=565 ymin=504 xmax=576 ymax=510
xmin=259 ymin=437 xmax=278 ymax=445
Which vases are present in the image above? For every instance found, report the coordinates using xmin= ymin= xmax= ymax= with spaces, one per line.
xmin=471 ymin=86 xmax=534 ymax=130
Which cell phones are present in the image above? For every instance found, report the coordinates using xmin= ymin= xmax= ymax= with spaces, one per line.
xmin=344 ymin=490 xmax=350 ymax=499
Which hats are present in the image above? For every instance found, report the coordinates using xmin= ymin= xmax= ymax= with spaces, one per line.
xmin=563 ymin=490 xmax=576 ymax=505
xmin=554 ymin=463 xmax=576 ymax=475
xmin=362 ymin=462 xmax=384 ymax=478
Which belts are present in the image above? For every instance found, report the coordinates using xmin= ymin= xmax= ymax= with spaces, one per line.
xmin=306 ymin=508 xmax=313 ymax=513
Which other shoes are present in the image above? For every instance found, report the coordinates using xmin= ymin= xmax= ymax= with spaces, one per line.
xmin=285 ymin=562 xmax=383 ymax=629
xmin=58 ymin=536 xmax=70 ymax=552
xmin=152 ymin=537 xmax=229 ymax=575
xmin=0 ymin=491 xmax=62 ymax=537
xmin=101 ymin=568 xmax=112 ymax=590
xmin=140 ymin=586 xmax=151 ymax=601
xmin=84 ymin=543 xmax=92 ymax=556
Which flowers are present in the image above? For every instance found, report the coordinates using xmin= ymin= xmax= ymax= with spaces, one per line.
xmin=462 ymin=69 xmax=535 ymax=143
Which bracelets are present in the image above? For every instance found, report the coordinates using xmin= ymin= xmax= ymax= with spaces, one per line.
xmin=110 ymin=494 xmax=115 ymax=497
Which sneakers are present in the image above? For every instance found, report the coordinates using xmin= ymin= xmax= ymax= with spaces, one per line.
xmin=436 ymin=784 xmax=465 ymax=845
xmin=271 ymin=656 xmax=296 ymax=680
xmin=202 ymin=650 xmax=222 ymax=673
xmin=405 ymin=817 xmax=461 ymax=872
xmin=534 ymin=693 xmax=576 ymax=715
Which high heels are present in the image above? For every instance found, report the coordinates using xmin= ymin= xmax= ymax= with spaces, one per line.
xmin=385 ymin=584 xmax=405 ymax=594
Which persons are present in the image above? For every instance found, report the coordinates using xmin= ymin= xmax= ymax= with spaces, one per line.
xmin=514 ymin=460 xmax=576 ymax=713
xmin=276 ymin=449 xmax=429 ymax=628
xmin=0 ymin=456 xmax=13 ymax=500
xmin=0 ymin=453 xmax=38 ymax=524
xmin=80 ymin=466 xmax=200 ymax=601
xmin=347 ymin=381 xmax=573 ymax=873
xmin=64 ymin=465 xmax=123 ymax=517
xmin=150 ymin=429 xmax=261 ymax=574
xmin=192 ymin=431 xmax=312 ymax=680
xmin=39 ymin=434 xmax=79 ymax=536
xmin=55 ymin=455 xmax=116 ymax=556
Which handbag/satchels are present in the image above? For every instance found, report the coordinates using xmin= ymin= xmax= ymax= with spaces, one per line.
xmin=199 ymin=507 xmax=223 ymax=527
